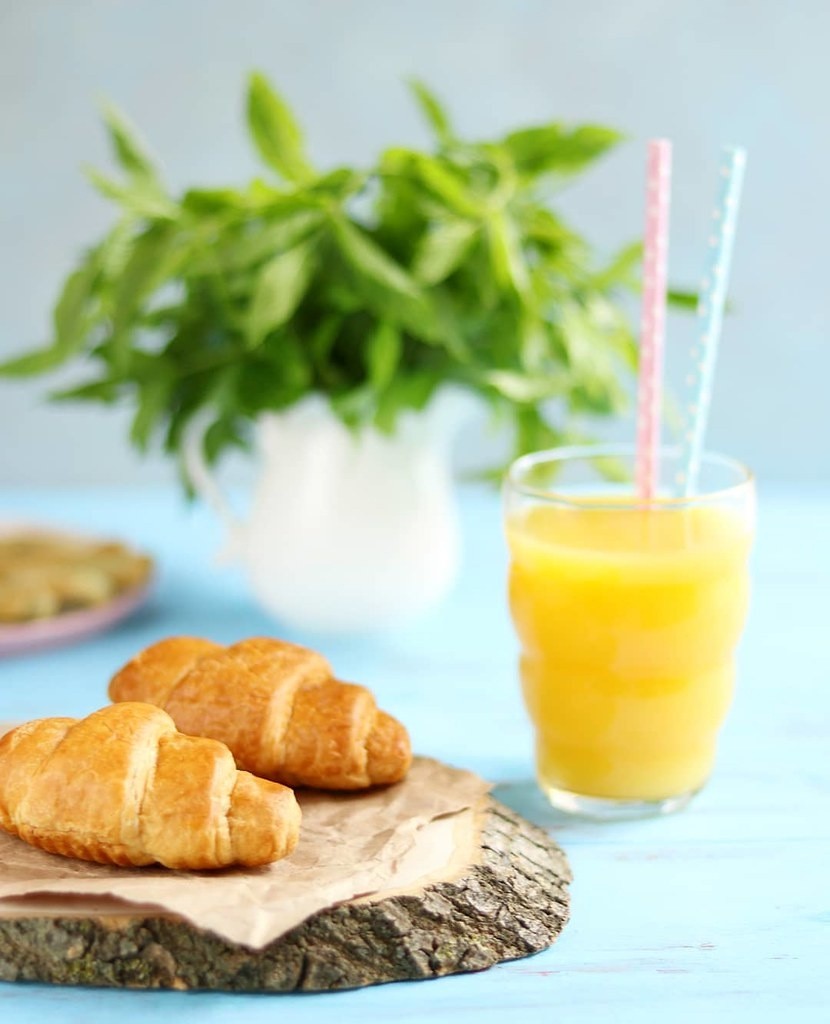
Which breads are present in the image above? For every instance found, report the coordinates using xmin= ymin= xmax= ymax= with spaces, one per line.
xmin=103 ymin=634 xmax=412 ymax=789
xmin=0 ymin=702 xmax=310 ymax=871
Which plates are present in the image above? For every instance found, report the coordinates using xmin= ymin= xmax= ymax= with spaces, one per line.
xmin=0 ymin=519 xmax=156 ymax=656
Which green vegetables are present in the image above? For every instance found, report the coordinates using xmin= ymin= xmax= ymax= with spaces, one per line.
xmin=2 ymin=75 xmax=711 ymax=492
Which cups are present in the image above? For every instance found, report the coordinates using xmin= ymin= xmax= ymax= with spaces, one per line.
xmin=501 ymin=443 xmax=761 ymax=821
